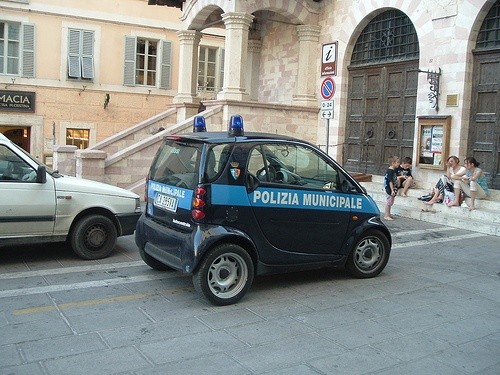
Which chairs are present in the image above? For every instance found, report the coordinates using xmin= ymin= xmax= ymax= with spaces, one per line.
xmin=207 ymin=145 xmax=230 ymax=179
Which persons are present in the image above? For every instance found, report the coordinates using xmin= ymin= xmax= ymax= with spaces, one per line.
xmin=398 ymin=156 xmax=415 ymax=198
xmin=451 ymin=158 xmax=490 ymax=211
xmin=424 ymin=156 xmax=466 ymax=206
xmin=383 ymin=154 xmax=402 ymax=221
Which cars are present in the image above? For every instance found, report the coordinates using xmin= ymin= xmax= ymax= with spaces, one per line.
xmin=136 ymin=115 xmax=392 ymax=305
xmin=0 ymin=132 xmax=144 ymax=260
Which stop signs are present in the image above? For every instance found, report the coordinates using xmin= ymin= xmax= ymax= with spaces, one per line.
xmin=321 ymin=78 xmax=336 ymax=100
